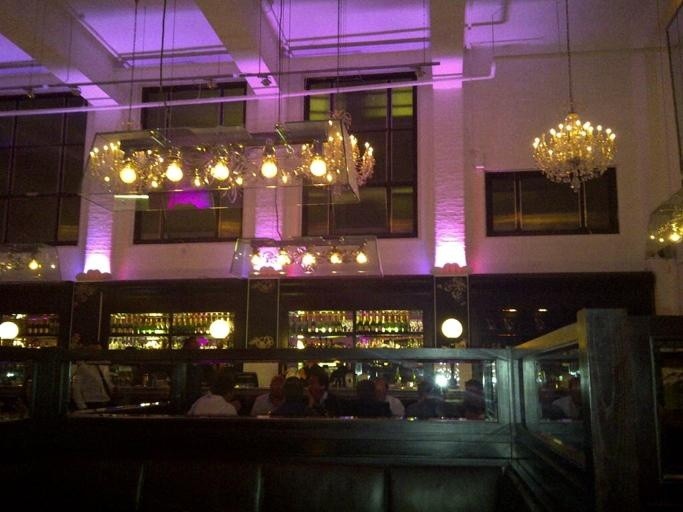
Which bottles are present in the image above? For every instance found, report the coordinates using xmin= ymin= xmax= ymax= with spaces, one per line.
xmin=170 ymin=335 xmax=234 ymax=350
xmin=109 ymin=312 xmax=235 ymax=335
xmin=354 ymin=310 xmax=423 ymax=335
xmin=355 ymin=336 xmax=420 ymax=348
xmin=287 ymin=310 xmax=351 ymax=333
xmin=108 ymin=336 xmax=169 ymax=350
xmin=409 ymin=368 xmax=416 ymax=387
xmin=26 ymin=316 xmax=59 ymax=335
xmin=143 ymin=372 xmax=152 ymax=386
xmin=288 ymin=335 xmax=348 ymax=349
xmin=394 ymin=366 xmax=401 ymax=386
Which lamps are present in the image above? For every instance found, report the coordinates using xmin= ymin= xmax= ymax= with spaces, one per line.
xmin=441 ymin=318 xmax=463 ymax=389
xmin=1 ymin=1 xmax=63 ymax=280
xmin=76 ymin=2 xmax=383 ymax=215
xmin=532 ymin=0 xmax=618 ymax=193
xmin=223 ymin=1 xmax=384 ymax=281
xmin=0 ymin=321 xmax=19 ymax=347
xmin=210 ymin=320 xmax=230 ymax=349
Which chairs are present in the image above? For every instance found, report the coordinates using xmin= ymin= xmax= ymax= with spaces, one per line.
xmin=139 ymin=459 xmax=263 ymax=512
xmin=259 ymin=462 xmax=388 ymax=512
xmin=389 ymin=465 xmax=509 ymax=512
xmin=47 ymin=461 xmax=144 ymax=512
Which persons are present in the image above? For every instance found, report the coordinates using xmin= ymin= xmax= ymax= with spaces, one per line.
xmin=185 ymin=360 xmax=485 ymax=420
xmin=71 ymin=343 xmax=114 ymax=410
xmin=540 ymin=380 xmax=585 ymax=430
